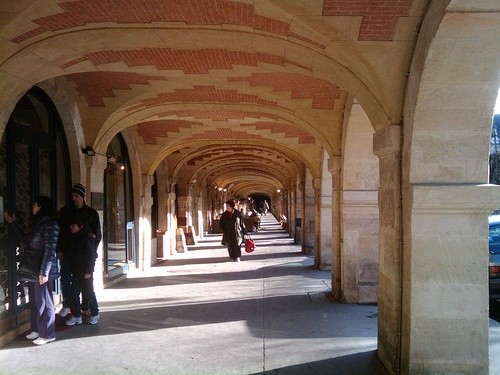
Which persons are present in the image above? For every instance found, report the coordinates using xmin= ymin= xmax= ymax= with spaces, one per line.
xmin=3 ymin=195 xmax=61 ymax=346
xmin=237 ymin=197 xmax=287 ymax=231
xmin=54 ymin=183 xmax=102 ymax=317
xmin=219 ymin=200 xmax=249 ymax=263
xmin=60 ymin=215 xmax=100 ymax=326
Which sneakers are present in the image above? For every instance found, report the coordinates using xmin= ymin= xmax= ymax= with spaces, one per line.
xmin=88 ymin=314 xmax=100 ymax=324
xmin=65 ymin=316 xmax=82 ymax=326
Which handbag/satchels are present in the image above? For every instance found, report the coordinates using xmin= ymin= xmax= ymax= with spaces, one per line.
xmin=16 ymin=249 xmax=43 ymax=279
xmin=244 ymin=234 xmax=254 ymax=253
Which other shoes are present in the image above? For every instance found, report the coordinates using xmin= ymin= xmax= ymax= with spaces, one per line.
xmin=59 ymin=308 xmax=71 ymax=317
xmin=26 ymin=332 xmax=39 ymax=340
xmin=235 ymin=257 xmax=240 ymax=262
xmin=230 ymin=258 xmax=234 ymax=262
xmin=32 ymin=337 xmax=56 ymax=346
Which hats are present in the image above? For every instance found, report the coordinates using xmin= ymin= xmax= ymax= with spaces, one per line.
xmin=71 ymin=183 xmax=85 ymax=198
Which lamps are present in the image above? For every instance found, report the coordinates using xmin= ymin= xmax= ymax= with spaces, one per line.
xmin=82 ymin=146 xmax=95 ymax=156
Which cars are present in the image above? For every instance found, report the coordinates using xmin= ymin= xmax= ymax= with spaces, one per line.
xmin=489 ymin=215 xmax=500 ymax=310
